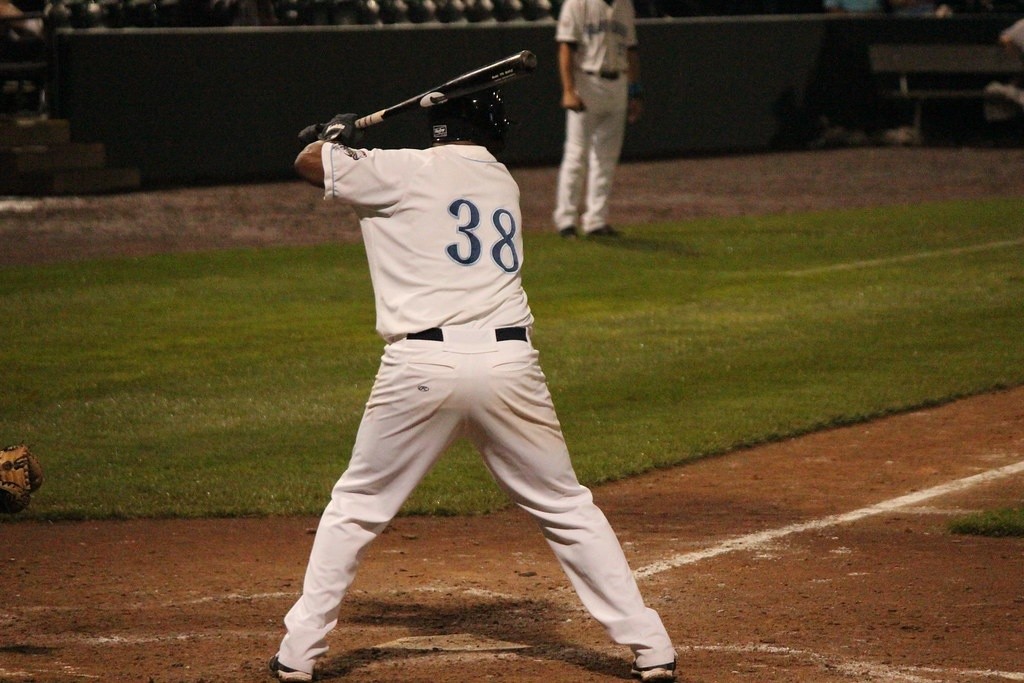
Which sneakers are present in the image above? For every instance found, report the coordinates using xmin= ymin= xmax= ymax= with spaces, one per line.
xmin=270 ymin=656 xmax=315 ymax=679
xmin=632 ymin=660 xmax=677 ymax=681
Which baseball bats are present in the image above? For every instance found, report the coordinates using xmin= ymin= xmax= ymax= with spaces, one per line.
xmin=298 ymin=52 xmax=537 ymax=143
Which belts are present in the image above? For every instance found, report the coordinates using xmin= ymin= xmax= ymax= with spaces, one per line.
xmin=408 ymin=323 xmax=527 ymax=341
xmin=600 ymin=69 xmax=621 ymax=80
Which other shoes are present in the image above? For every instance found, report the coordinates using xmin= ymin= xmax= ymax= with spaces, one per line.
xmin=559 ymin=225 xmax=625 ymax=242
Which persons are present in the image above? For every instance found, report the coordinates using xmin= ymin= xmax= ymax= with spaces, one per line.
xmin=553 ymin=0 xmax=639 ymax=238
xmin=267 ymin=86 xmax=679 ymax=683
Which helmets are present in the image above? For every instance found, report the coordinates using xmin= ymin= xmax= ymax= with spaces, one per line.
xmin=433 ymin=63 xmax=528 ymax=152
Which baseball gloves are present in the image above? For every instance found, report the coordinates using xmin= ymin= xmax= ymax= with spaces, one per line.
xmin=0 ymin=443 xmax=43 ymax=514
